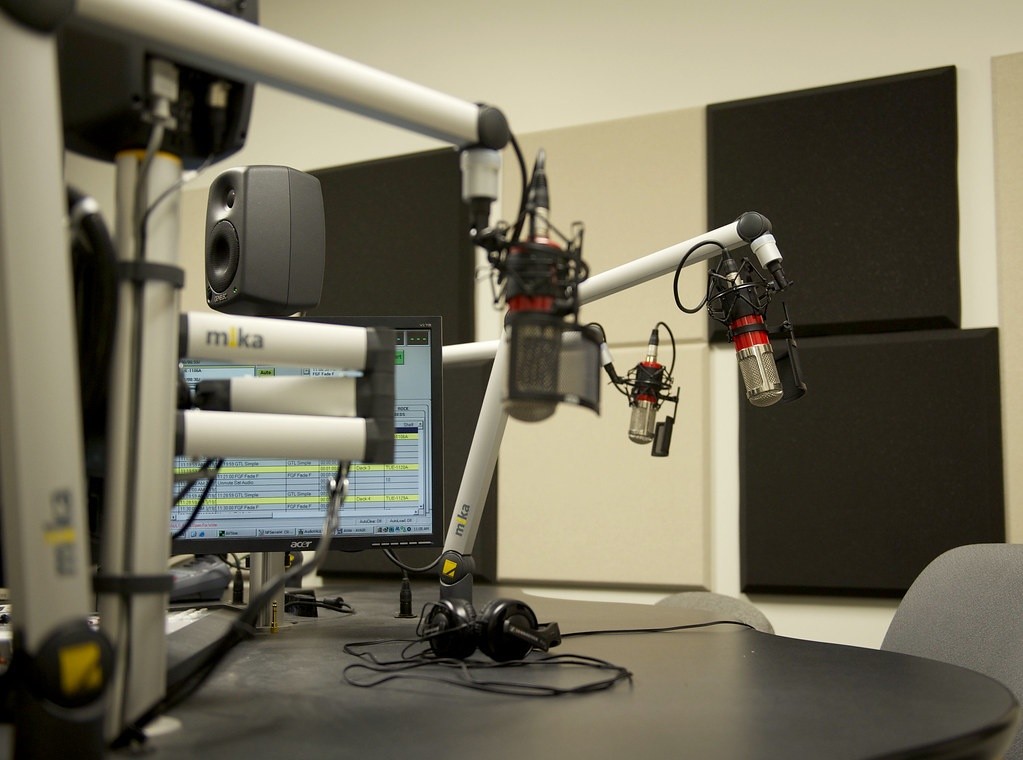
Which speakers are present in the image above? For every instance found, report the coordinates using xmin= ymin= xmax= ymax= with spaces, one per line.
xmin=62 ymin=0 xmax=260 ymax=166
xmin=204 ymin=165 xmax=327 ymax=318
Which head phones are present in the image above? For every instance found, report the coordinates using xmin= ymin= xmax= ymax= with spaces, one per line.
xmin=418 ymin=599 xmax=561 ymax=662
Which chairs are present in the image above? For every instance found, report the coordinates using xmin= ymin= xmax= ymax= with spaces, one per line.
xmin=880 ymin=543 xmax=1022 ymax=760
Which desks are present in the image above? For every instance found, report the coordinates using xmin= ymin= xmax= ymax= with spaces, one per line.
xmin=112 ymin=579 xmax=1018 ymax=759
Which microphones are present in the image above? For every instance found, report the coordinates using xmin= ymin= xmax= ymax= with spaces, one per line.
xmin=721 ymin=248 xmax=784 ymax=408
xmin=629 ymin=328 xmax=663 ymax=444
xmin=503 ymin=168 xmax=565 ymax=422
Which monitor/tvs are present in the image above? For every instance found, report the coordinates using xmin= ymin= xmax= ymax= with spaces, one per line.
xmin=168 ymin=313 xmax=445 ymax=554
